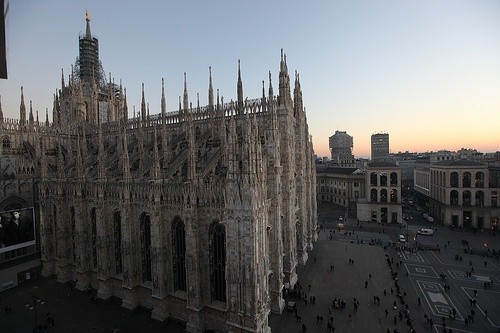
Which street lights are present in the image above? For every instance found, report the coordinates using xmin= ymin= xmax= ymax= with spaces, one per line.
xmin=25 ymin=296 xmax=44 ymax=332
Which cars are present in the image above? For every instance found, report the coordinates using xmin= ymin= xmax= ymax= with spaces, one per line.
xmin=316 ymin=195 xmax=438 ymax=246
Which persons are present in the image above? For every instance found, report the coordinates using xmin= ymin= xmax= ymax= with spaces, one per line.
xmin=348 ymin=258 xmax=351 ymax=264
xmin=308 ymin=284 xmax=311 ymax=289
xmin=474 ymin=289 xmax=477 ymax=297
xmin=377 ymin=296 xmax=380 ymax=305
xmin=8 ymin=307 xmax=11 ymax=312
xmin=5 ymin=305 xmax=8 ymax=312
xmin=294 ymin=284 xmax=296 ymax=290
xmin=469 ymin=299 xmax=472 ymax=306
xmin=332 ymin=264 xmax=335 ymax=271
xmin=315 ymin=257 xmax=316 ymax=263
xmin=386 ymin=328 xmax=390 ymax=333
xmin=31 ymin=311 xmax=55 ymax=333
xmin=369 ymin=273 xmax=372 ymax=279
xmin=384 ymin=289 xmax=387 ymax=296
xmin=484 ymin=310 xmax=488 ymax=315
xmin=320 ymin=314 xmax=323 ymax=323
xmin=394 ymin=289 xmax=457 ymax=333
xmin=2 ymin=210 xmax=29 ymax=249
xmin=316 ymin=314 xmax=319 ymax=323
xmin=386 ymin=259 xmax=495 ymax=288
xmin=327 ymin=294 xmax=360 ymax=333
xmin=473 ymin=299 xmax=476 ymax=306
xmin=316 ymin=218 xmax=500 ymax=258
xmin=285 ymin=288 xmax=316 ymax=323
xmin=391 ymin=287 xmax=394 ymax=295
xmin=296 ymin=280 xmax=299 ymax=287
xmin=465 ymin=309 xmax=475 ymax=326
xmin=302 ymin=324 xmax=306 ymax=333
xmin=88 ymin=286 xmax=92 ymax=295
xmin=352 ymin=259 xmax=354 ymax=264
xmin=385 ymin=308 xmax=388 ymax=315
xmin=373 ymin=296 xmax=376 ymax=304
xmin=365 ymin=281 xmax=368 ymax=288
xmin=330 ymin=265 xmax=332 ymax=271
